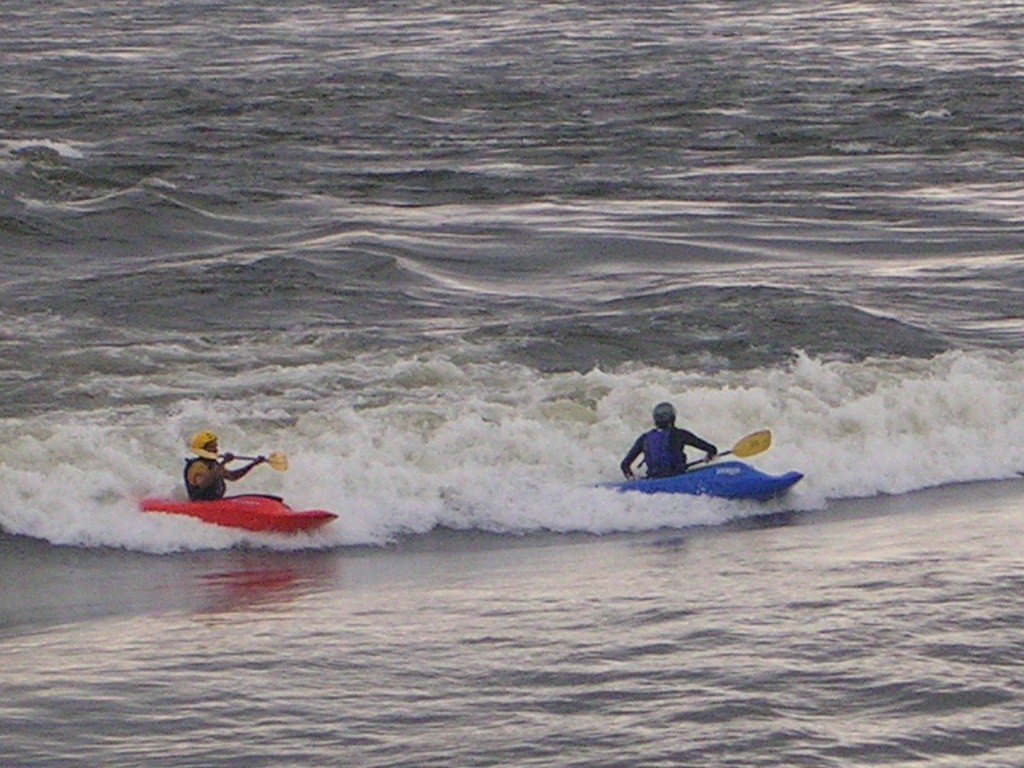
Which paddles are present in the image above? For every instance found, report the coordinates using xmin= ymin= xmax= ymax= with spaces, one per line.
xmin=188 ymin=442 xmax=289 ymax=475
xmin=641 ymin=429 xmax=771 ymax=480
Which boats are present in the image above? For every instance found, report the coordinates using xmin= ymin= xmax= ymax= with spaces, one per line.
xmin=139 ymin=492 xmax=341 ymax=533
xmin=590 ymin=460 xmax=806 ymax=502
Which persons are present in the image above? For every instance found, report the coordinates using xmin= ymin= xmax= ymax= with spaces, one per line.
xmin=620 ymin=401 xmax=717 ymax=481
xmin=183 ymin=430 xmax=266 ymax=500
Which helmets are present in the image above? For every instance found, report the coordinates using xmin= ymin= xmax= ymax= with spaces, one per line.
xmin=191 ymin=431 xmax=218 ymax=459
xmin=654 ymin=403 xmax=673 ymax=423
